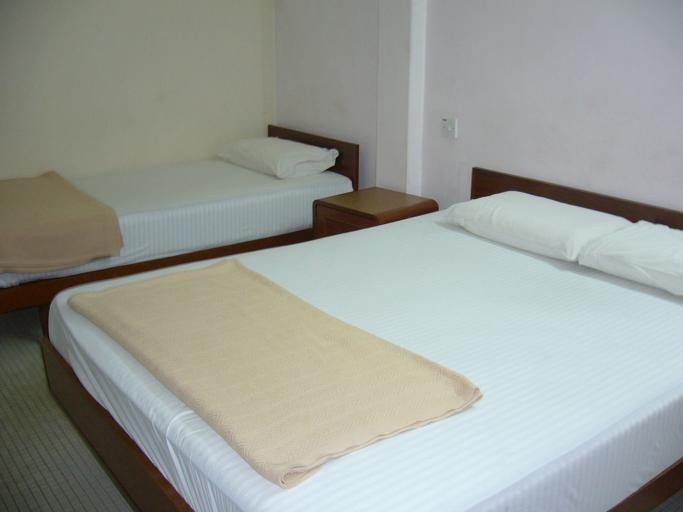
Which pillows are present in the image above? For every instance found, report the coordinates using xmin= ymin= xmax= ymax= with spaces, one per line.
xmin=217 ymin=136 xmax=339 ymax=179
xmin=444 ymin=191 xmax=632 ymax=261
xmin=578 ymin=220 xmax=683 ymax=299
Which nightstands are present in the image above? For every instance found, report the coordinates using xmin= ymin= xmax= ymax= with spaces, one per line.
xmin=313 ymin=186 xmax=439 ymax=240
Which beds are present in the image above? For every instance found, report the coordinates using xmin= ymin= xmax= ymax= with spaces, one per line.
xmin=43 ymin=167 xmax=683 ymax=511
xmin=0 ymin=124 xmax=360 ymax=314
